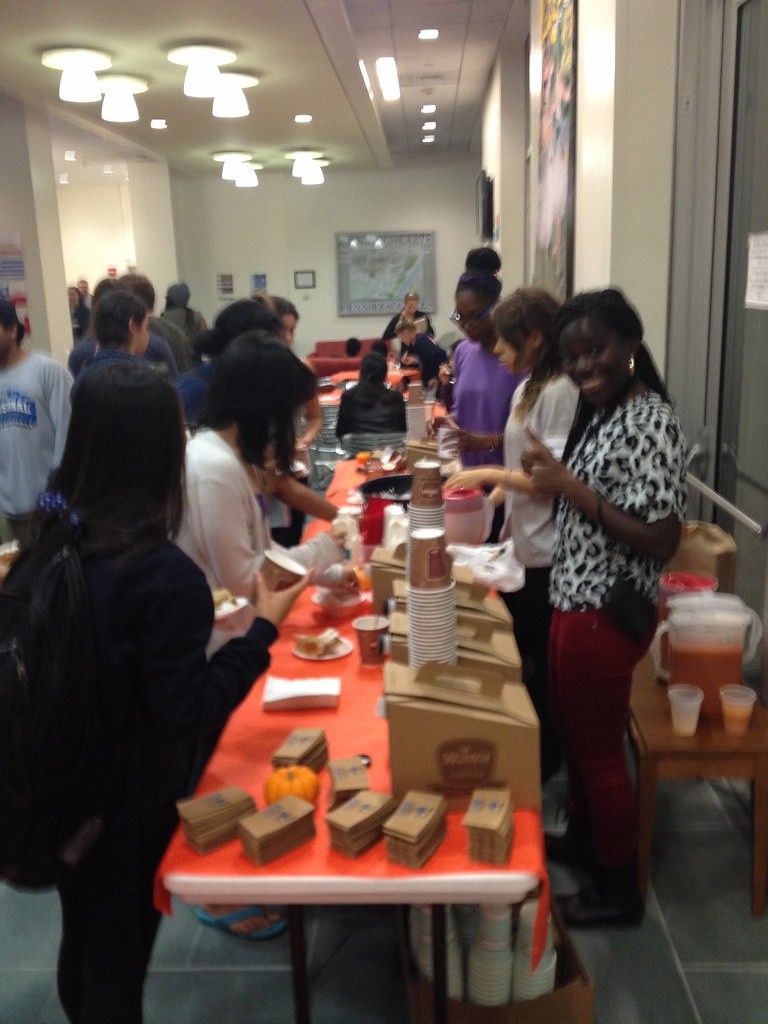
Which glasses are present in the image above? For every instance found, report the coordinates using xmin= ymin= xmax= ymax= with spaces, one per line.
xmin=448 ymin=302 xmax=495 ymax=337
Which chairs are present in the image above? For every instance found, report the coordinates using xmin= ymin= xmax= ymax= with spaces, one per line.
xmin=302 ymin=405 xmax=345 ymax=491
xmin=341 ymin=432 xmax=410 ymax=460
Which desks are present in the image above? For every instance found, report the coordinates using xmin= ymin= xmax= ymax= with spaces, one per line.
xmin=628 ymin=653 xmax=768 ymax=918
xmin=153 ymin=458 xmax=551 ymax=1024
xmin=317 ymin=370 xmax=448 ymax=417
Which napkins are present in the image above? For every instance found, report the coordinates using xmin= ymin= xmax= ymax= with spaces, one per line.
xmin=261 ymin=673 xmax=342 ymax=712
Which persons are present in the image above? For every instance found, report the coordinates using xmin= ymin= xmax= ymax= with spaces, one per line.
xmin=0 ymin=352 xmax=314 ymax=1024
xmin=0 ymin=248 xmax=688 ymax=940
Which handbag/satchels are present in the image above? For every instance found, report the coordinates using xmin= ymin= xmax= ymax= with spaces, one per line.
xmin=665 ymin=519 xmax=739 ymax=598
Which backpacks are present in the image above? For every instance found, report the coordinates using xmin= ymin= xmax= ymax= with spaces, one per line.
xmin=0 ymin=516 xmax=172 ymax=892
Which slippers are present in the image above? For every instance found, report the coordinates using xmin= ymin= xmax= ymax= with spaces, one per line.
xmin=192 ymin=903 xmax=289 ymax=943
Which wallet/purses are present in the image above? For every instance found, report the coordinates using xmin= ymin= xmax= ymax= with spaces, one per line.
xmin=600 ymin=577 xmax=657 ymax=642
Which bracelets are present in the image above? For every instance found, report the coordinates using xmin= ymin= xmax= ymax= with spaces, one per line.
xmin=487 ymin=432 xmax=502 ymax=452
xmin=596 ymin=492 xmax=605 ymax=522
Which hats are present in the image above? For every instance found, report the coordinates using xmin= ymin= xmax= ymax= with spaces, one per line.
xmin=167 ymin=284 xmax=190 ymax=307
xmin=0 ymin=298 xmax=25 ymax=342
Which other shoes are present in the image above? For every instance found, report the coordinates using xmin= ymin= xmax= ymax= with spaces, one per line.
xmin=555 ymin=866 xmax=647 ymax=927
xmin=544 ymin=832 xmax=592 ymax=874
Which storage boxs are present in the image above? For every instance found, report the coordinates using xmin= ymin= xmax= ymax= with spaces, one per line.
xmin=389 ymin=610 xmax=523 ymax=680
xmin=369 ymin=547 xmax=472 ymax=615
xmin=391 ymin=578 xmax=512 ymax=631
xmin=380 ymin=662 xmax=542 ymax=812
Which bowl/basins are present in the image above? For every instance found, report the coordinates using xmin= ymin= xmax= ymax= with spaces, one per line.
xmin=311 ymin=591 xmax=367 ymax=618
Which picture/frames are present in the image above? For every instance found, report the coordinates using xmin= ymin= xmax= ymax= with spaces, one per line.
xmin=334 ymin=230 xmax=438 ymax=316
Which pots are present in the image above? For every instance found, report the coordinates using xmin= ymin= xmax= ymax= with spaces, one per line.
xmin=353 ymin=472 xmax=453 ymax=508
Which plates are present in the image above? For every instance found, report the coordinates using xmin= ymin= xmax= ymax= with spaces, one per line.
xmin=212 ymin=594 xmax=249 ymax=624
xmin=291 ymin=638 xmax=353 ymax=660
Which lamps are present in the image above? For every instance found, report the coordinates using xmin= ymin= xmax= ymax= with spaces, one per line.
xmin=234 ymin=161 xmax=264 ymax=188
xmin=41 ymin=48 xmax=114 ymax=102
xmin=99 ymin=74 xmax=149 ymax=123
xmin=212 ymin=72 xmax=259 ymax=118
xmin=212 ymin=151 xmax=252 ymax=181
xmin=167 ymin=44 xmax=237 ymax=98
xmin=301 ymin=159 xmax=330 ymax=185
xmin=286 ymin=150 xmax=324 ymax=178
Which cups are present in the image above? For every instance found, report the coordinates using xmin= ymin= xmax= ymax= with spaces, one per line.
xmin=718 ymin=685 xmax=757 ymax=737
xmin=405 ymin=383 xmax=428 ymax=441
xmin=246 ymin=550 xmax=308 ymax=607
xmin=405 ymin=456 xmax=459 ymax=670
xmin=666 ymin=683 xmax=704 ymax=737
xmin=352 ymin=615 xmax=392 ymax=664
xmin=409 ymin=900 xmax=556 ymax=1005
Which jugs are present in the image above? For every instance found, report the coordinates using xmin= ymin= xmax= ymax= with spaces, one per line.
xmin=652 ymin=570 xmax=763 ymax=720
xmin=439 ymin=483 xmax=485 ymax=547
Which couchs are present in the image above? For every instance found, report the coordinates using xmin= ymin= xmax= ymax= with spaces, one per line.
xmin=305 ymin=338 xmax=400 ymax=378
